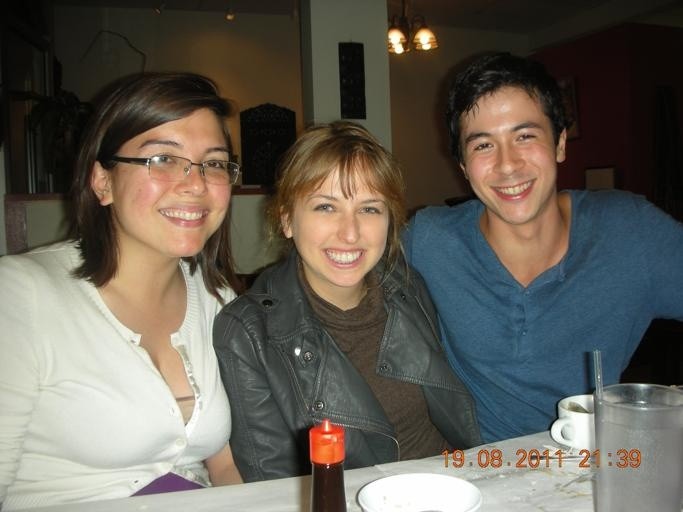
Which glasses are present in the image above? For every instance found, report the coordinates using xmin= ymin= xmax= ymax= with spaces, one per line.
xmin=100 ymin=152 xmax=244 ymax=187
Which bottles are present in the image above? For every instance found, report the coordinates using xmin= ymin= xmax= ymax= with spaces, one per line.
xmin=309 ymin=419 xmax=346 ymax=508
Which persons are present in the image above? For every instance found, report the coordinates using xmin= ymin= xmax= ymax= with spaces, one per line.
xmin=404 ymin=50 xmax=683 ymax=444
xmin=0 ymin=70 xmax=242 ymax=512
xmin=213 ymin=119 xmax=484 ymax=487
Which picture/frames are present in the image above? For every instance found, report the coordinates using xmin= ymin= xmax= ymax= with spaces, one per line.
xmin=555 ymin=79 xmax=581 ymax=146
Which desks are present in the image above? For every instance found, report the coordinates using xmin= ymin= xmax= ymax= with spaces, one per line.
xmin=27 ymin=429 xmax=597 ymax=511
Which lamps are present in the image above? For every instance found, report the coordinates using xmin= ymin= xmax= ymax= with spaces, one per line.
xmin=152 ymin=1 xmax=167 ymax=16
xmin=224 ymin=0 xmax=236 ymax=22
xmin=386 ymin=5 xmax=438 ymax=54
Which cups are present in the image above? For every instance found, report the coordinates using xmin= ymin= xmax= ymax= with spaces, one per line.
xmin=551 ymin=394 xmax=595 ymax=454
xmin=594 ymin=383 xmax=683 ymax=512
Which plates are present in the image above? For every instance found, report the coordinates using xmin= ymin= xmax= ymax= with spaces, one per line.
xmin=357 ymin=473 xmax=483 ymax=512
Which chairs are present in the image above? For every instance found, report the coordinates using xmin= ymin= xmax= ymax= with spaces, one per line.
xmin=5 ymin=191 xmax=74 ymax=253
xmin=225 ymin=186 xmax=290 ymax=274
